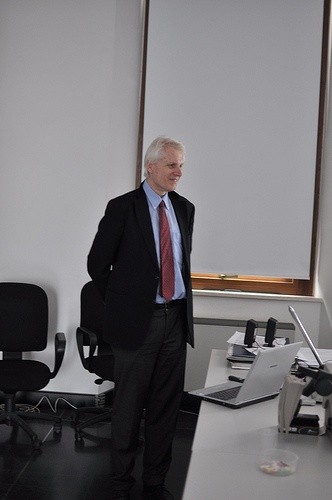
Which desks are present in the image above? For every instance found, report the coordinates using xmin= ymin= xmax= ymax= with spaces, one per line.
xmin=182 ymin=348 xmax=332 ymax=500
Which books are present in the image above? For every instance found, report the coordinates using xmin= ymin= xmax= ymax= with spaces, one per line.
xmin=225 ymin=330 xmax=287 ymax=371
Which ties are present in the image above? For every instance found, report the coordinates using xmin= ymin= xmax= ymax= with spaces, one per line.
xmin=158 ymin=200 xmax=175 ymax=302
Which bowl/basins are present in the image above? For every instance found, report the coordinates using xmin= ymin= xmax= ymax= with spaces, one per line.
xmin=259 ymin=448 xmax=299 ymax=475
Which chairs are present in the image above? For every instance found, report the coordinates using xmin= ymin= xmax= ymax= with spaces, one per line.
xmin=71 ymin=281 xmax=116 ymax=446
xmin=0 ymin=282 xmax=66 ymax=450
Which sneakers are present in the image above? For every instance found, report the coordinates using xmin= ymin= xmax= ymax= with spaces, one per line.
xmin=111 ymin=484 xmax=177 ymax=500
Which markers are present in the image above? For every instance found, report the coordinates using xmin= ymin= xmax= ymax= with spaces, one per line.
xmin=228 ymin=375 xmax=245 ymax=383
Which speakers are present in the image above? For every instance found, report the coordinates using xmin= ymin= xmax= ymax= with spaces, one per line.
xmin=262 ymin=317 xmax=278 ymax=347
xmin=244 ymin=319 xmax=258 ymax=347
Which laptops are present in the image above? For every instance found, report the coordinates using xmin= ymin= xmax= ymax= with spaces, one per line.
xmin=188 ymin=342 xmax=303 ymax=408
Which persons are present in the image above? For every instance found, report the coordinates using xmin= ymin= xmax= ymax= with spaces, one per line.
xmin=85 ymin=137 xmax=196 ymax=496
